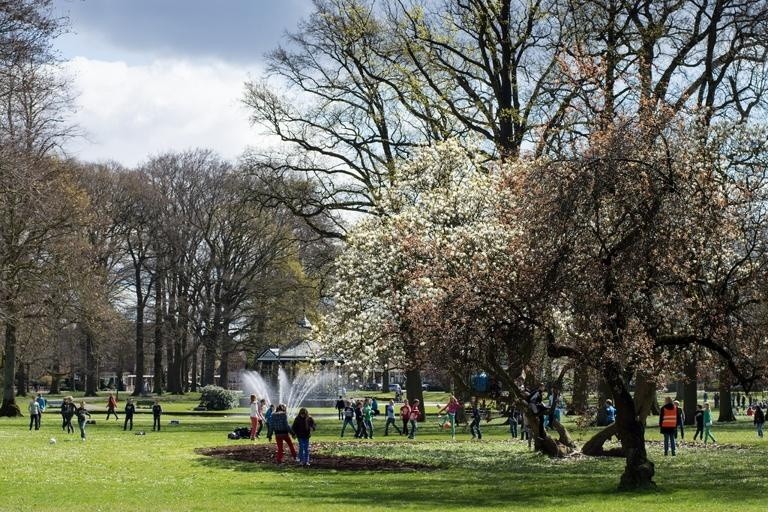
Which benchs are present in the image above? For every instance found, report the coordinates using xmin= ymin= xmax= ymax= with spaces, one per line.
xmin=466 ymin=407 xmax=491 ymax=422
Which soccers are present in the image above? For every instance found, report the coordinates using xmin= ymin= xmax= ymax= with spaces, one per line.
xmin=444 ymin=420 xmax=452 ymax=431
xmin=48 ymin=437 xmax=56 ymax=443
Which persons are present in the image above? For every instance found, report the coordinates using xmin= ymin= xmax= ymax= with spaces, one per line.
xmin=336 ymin=388 xmax=421 ymax=439
xmin=60 ymin=397 xmax=68 ymax=430
xmin=36 ymin=394 xmax=46 ymax=428
xmin=76 ymin=402 xmax=91 ymax=440
xmin=121 ymin=399 xmax=135 ymax=430
xmin=105 ymin=393 xmax=119 ymax=421
xmin=250 ymin=394 xmax=316 ymax=467
xmin=660 ymin=392 xmax=768 ymax=456
xmin=439 ymin=382 xmax=566 ymax=447
xmin=66 ymin=396 xmax=77 ymax=433
xmin=605 ymin=398 xmax=621 ymax=442
xmin=28 ymin=395 xmax=40 ymax=430
xmin=151 ymin=399 xmax=162 ymax=431
